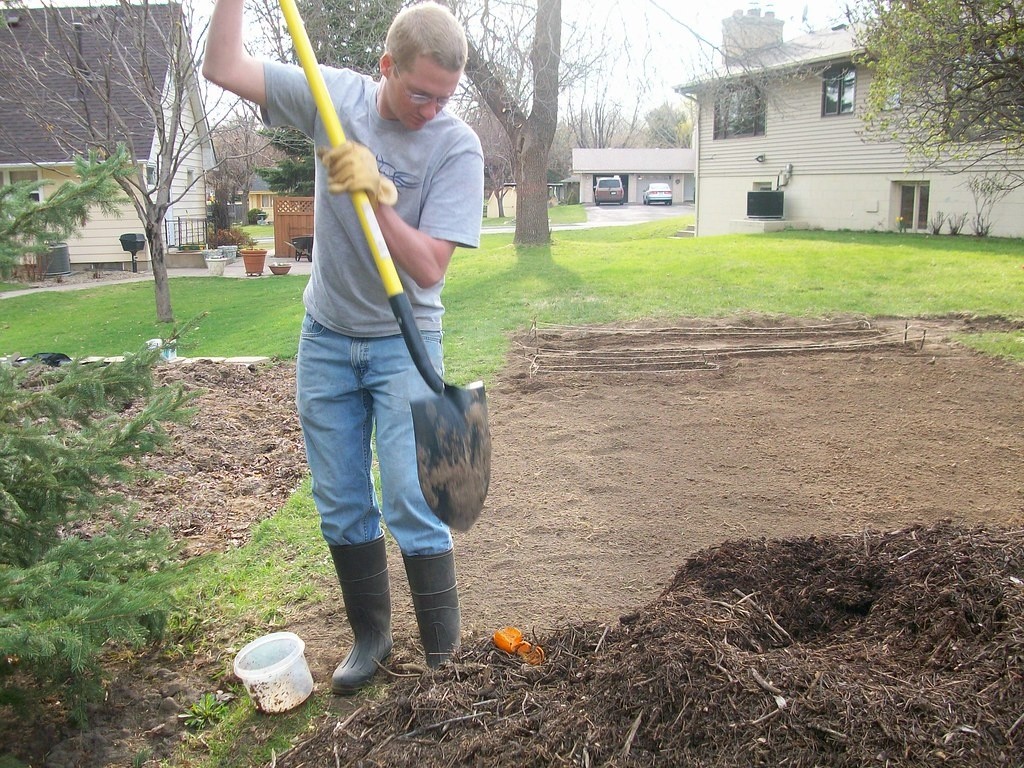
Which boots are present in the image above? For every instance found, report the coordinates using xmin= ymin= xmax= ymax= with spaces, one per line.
xmin=331 ymin=528 xmax=393 ymax=695
xmin=402 ymin=546 xmax=460 ymax=668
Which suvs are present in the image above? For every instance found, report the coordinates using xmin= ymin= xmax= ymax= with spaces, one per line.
xmin=593 ymin=177 xmax=626 ymax=206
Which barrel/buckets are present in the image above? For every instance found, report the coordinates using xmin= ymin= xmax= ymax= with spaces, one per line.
xmin=233 ymin=632 xmax=314 ymax=713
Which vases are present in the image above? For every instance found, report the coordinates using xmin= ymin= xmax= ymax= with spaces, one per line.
xmin=269 ymin=264 xmax=292 ymax=274
xmin=207 ymin=257 xmax=227 ymax=276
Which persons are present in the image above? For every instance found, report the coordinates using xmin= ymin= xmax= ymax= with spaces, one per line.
xmin=201 ymin=0 xmax=487 ymax=697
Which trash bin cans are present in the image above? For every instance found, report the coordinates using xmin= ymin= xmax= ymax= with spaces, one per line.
xmin=35 ymin=232 xmax=72 ymax=276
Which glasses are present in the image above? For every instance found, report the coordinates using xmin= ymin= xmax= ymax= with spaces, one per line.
xmin=392 ymin=61 xmax=452 ymax=107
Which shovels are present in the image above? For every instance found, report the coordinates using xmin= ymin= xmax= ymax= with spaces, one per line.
xmin=276 ymin=0 xmax=496 ymax=537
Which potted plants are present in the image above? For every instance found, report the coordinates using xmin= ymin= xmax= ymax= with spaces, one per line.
xmin=200 ymin=222 xmax=259 ymax=268
xmin=239 ymin=239 xmax=267 ymax=276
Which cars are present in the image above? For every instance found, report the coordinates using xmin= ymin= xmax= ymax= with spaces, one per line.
xmin=643 ymin=182 xmax=672 ymax=205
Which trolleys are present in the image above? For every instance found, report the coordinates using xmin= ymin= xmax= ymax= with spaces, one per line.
xmin=283 ymin=235 xmax=313 ymax=263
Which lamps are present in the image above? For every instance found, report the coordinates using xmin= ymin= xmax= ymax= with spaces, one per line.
xmin=756 ymin=156 xmax=763 ymax=162
